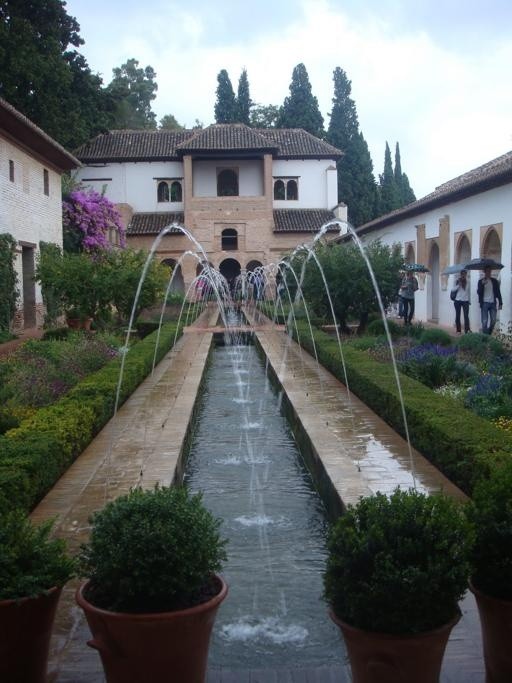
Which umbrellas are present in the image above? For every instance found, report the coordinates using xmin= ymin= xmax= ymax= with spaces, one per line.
xmin=400 ymin=263 xmax=430 ymax=272
xmin=441 ymin=262 xmax=471 ymax=276
xmin=463 ymin=257 xmax=505 ymax=275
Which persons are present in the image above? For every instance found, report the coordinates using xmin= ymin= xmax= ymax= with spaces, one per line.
xmin=476 ymin=267 xmax=503 ymax=334
xmin=448 ymin=269 xmax=473 ymax=335
xmin=400 ymin=270 xmax=418 ymax=324
xmin=396 ymin=269 xmax=405 ymax=318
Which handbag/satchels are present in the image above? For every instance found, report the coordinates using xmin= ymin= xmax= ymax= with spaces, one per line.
xmin=450 ymin=291 xmax=456 ymax=299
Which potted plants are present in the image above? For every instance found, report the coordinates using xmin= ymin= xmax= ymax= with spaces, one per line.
xmin=0 ymin=509 xmax=80 ymax=683
xmin=466 ymin=461 xmax=512 ymax=683
xmin=322 ymin=491 xmax=476 ymax=683
xmin=32 ymin=243 xmax=116 ymax=331
xmin=74 ymin=485 xmax=228 ymax=683
